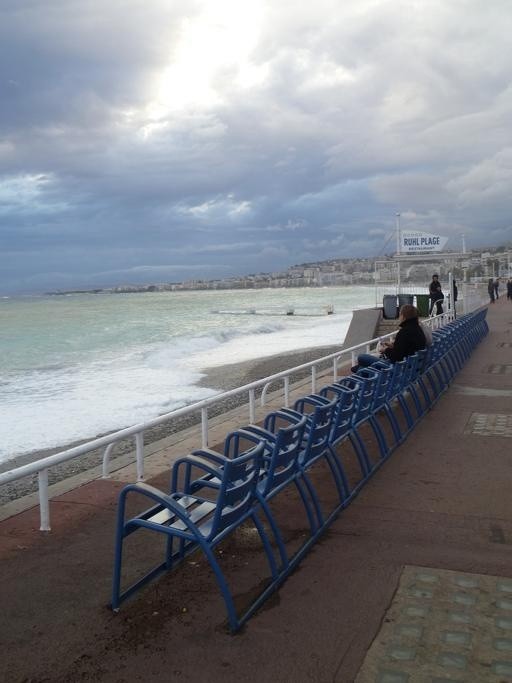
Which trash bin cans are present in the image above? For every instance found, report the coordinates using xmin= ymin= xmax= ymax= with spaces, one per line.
xmin=383 ymin=294 xmax=431 ymax=320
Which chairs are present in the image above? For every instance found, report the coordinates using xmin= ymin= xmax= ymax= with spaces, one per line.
xmin=177 ymin=414 xmax=315 ymax=583
xmin=300 ymin=308 xmax=491 ymax=503
xmin=240 ymin=396 xmax=348 ymax=538
xmin=105 ymin=439 xmax=279 ymax=637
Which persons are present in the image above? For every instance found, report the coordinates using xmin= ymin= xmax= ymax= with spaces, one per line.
xmin=434 ymin=285 xmax=444 ymax=315
xmin=390 ymin=308 xmax=432 ymax=346
xmin=488 ymin=279 xmax=495 ymax=302
xmin=358 ymin=305 xmax=425 ymax=365
xmin=494 ymin=279 xmax=499 ymax=299
xmin=510 ymin=277 xmax=512 ymax=300
xmin=506 ymin=280 xmax=511 ymax=297
xmin=429 ymin=275 xmax=441 ymax=316
xmin=447 ymin=279 xmax=457 ymax=319
